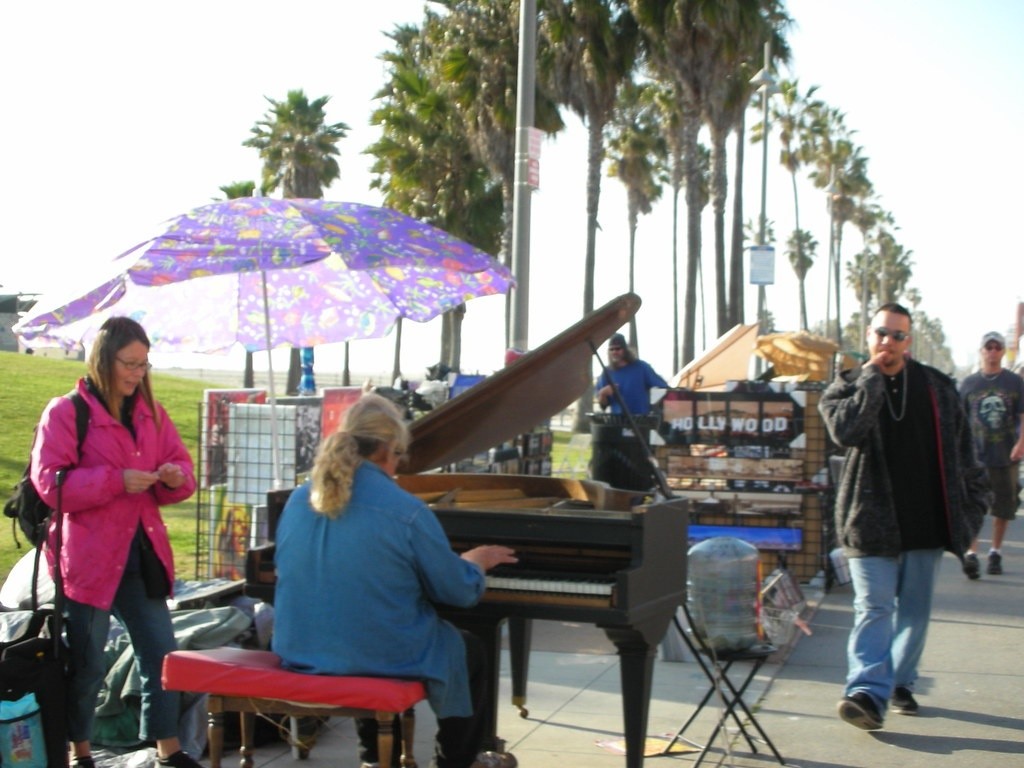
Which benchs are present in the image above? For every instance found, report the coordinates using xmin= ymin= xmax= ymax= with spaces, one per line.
xmin=161 ymin=646 xmax=428 ymax=768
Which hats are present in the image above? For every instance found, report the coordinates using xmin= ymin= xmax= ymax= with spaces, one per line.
xmin=610 ymin=334 xmax=625 ymax=346
xmin=981 ymin=331 xmax=1004 ymax=346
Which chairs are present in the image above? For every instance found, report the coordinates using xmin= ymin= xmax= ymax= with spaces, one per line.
xmin=662 ymin=603 xmax=785 ymax=768
xmin=553 ymin=433 xmax=593 ymax=480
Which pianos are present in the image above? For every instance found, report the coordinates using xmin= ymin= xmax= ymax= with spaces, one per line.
xmin=247 ymin=292 xmax=689 ymax=768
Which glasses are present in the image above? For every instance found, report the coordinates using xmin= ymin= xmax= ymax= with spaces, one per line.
xmin=609 ymin=347 xmax=622 ymax=351
xmin=116 ymin=357 xmax=152 ymax=371
xmin=874 ymin=326 xmax=908 ymax=341
xmin=984 ymin=345 xmax=1003 ymax=351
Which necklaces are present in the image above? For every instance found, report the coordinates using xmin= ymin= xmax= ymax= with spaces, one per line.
xmin=880 ymin=361 xmax=908 ymax=422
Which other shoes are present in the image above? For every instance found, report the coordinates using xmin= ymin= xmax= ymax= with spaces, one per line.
xmin=154 ymin=750 xmax=204 ymax=768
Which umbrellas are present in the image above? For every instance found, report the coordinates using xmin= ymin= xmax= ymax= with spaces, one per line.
xmin=8 ymin=189 xmax=521 ymax=487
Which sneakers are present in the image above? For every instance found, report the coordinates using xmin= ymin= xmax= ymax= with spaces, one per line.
xmin=360 ymin=762 xmax=378 ymax=768
xmin=837 ymin=693 xmax=883 ymax=731
xmin=429 ymin=747 xmax=517 ymax=768
xmin=986 ymin=552 xmax=1003 ymax=575
xmin=889 ymin=687 xmax=918 ymax=714
xmin=964 ymin=554 xmax=980 ymax=579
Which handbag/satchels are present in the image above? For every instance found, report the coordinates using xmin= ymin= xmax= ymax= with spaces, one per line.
xmin=3 ymin=476 xmax=49 ymax=549
xmin=0 ymin=615 xmax=71 ymax=702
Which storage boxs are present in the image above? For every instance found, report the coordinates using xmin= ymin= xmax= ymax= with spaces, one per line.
xmin=524 ymin=433 xmax=553 ymax=476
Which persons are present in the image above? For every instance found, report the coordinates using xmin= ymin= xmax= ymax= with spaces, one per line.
xmin=269 ymin=394 xmax=517 ymax=768
xmin=815 ymin=302 xmax=992 ymax=729
xmin=958 ymin=330 xmax=1024 ymax=579
xmin=28 ymin=314 xmax=205 ymax=768
xmin=595 ymin=333 xmax=668 ymax=445
